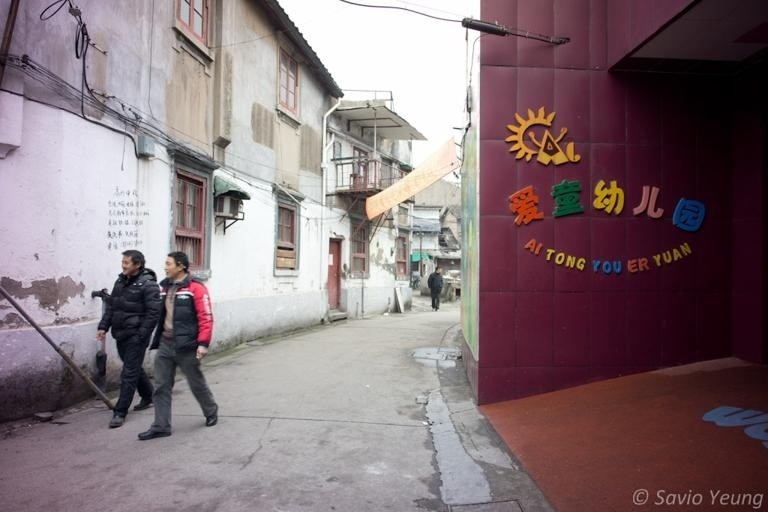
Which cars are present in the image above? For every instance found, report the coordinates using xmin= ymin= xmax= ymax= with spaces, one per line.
xmin=409 ymin=270 xmax=422 ymax=290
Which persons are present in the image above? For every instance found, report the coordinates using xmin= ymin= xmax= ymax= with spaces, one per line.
xmin=95 ymin=250 xmax=161 ymax=428
xmin=427 ymin=265 xmax=444 ymax=311
xmin=136 ymin=250 xmax=219 ymax=441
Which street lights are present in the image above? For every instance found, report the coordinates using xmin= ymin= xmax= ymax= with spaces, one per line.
xmin=414 ymin=232 xmax=425 ymax=276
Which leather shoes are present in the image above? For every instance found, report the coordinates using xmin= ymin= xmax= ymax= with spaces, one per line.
xmin=138 ymin=429 xmax=171 ymax=440
xmin=206 ymin=405 xmax=218 ymax=425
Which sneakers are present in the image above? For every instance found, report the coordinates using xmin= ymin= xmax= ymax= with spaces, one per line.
xmin=109 ymin=414 xmax=125 ymax=426
xmin=134 ymin=399 xmax=154 ymax=411
